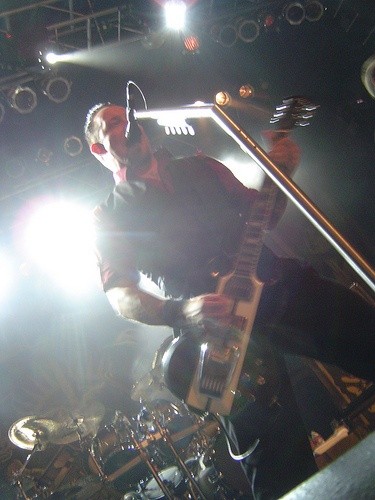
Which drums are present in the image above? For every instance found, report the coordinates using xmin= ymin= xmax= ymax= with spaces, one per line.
xmin=139 ymin=464 xmax=192 ymax=500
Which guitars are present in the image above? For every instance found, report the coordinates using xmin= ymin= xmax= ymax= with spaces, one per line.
xmin=160 ymin=93 xmax=318 ymax=420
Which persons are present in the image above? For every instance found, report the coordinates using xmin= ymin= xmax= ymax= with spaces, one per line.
xmin=85 ymin=101 xmax=373 ymax=499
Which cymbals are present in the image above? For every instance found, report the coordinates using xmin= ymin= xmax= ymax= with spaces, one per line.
xmin=42 ymin=400 xmax=106 ymax=445
xmin=8 ymin=414 xmax=56 ymax=451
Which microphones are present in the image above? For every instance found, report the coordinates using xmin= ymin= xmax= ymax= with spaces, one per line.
xmin=125 ymin=98 xmax=143 ymax=146
